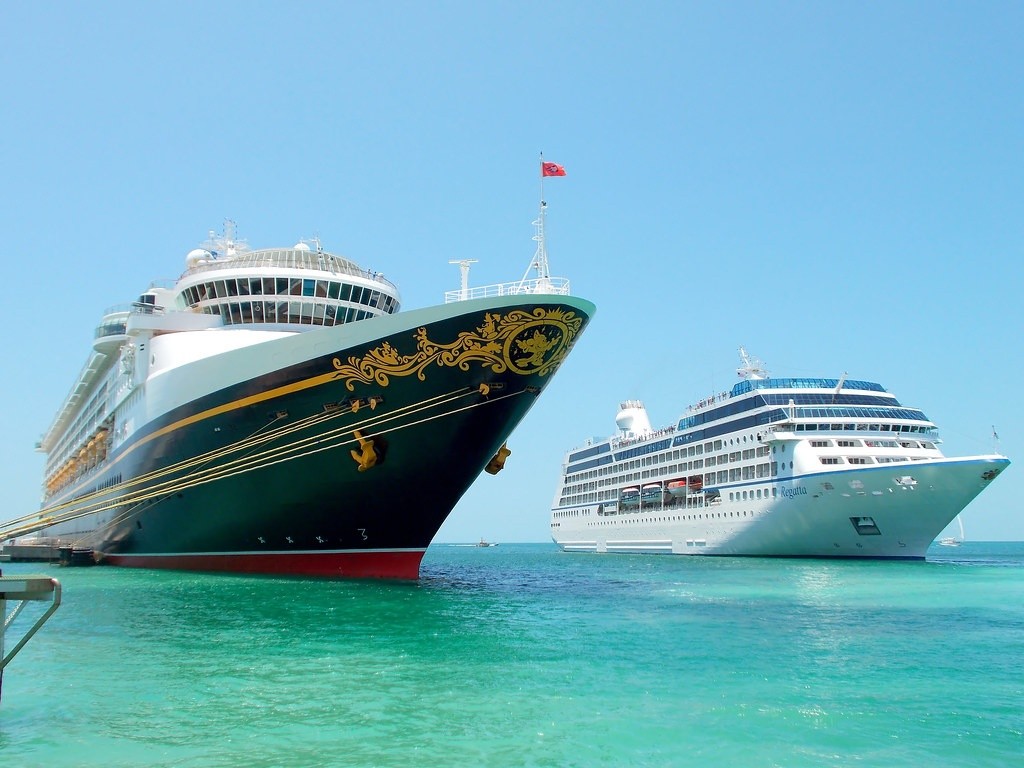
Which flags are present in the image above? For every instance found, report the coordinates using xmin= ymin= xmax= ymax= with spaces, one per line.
xmin=540 ymin=162 xmax=566 ymax=176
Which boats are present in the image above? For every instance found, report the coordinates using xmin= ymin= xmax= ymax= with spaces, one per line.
xmin=939 ymin=537 xmax=962 ymax=547
xmin=78 ymin=447 xmax=89 ymax=464
xmin=619 ymin=487 xmax=640 ymax=507
xmin=47 ymin=459 xmax=80 ymax=491
xmin=86 ymin=439 xmax=98 ymax=458
xmin=476 ymin=537 xmax=491 ymax=548
xmin=94 ymin=430 xmax=110 ymax=451
xmin=642 ymin=483 xmax=669 ymax=504
xmin=666 ymin=480 xmax=703 ymax=497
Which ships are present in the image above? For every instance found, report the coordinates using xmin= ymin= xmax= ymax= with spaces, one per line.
xmin=34 ymin=150 xmax=599 ymax=584
xmin=548 ymin=342 xmax=1013 ymax=560
